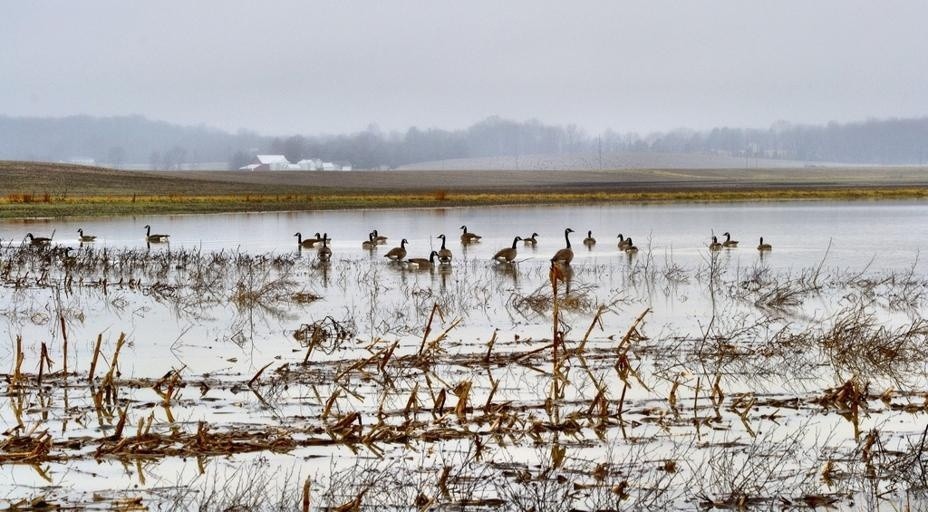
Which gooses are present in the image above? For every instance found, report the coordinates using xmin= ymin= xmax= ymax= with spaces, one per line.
xmin=361 ymin=225 xmax=640 ymax=267
xmin=77 ymin=228 xmax=97 ymax=241
xmin=293 ymin=231 xmax=334 ymax=262
xmin=25 ymin=232 xmax=53 ymax=244
xmin=143 ymin=224 xmax=170 ymax=242
xmin=709 ymin=236 xmax=721 ymax=250
xmin=723 ymin=232 xmax=739 ymax=246
xmin=757 ymin=236 xmax=772 ymax=250
